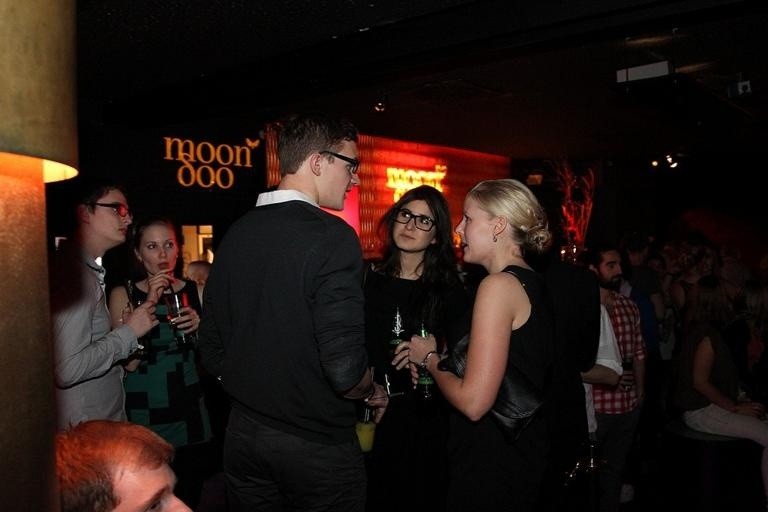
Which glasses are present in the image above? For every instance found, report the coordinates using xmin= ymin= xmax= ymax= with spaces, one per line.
xmin=391 ymin=205 xmax=436 ymax=232
xmin=93 ymin=202 xmax=130 ymax=216
xmin=320 ymin=150 xmax=361 ymax=173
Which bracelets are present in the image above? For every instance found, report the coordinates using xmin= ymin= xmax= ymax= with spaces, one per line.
xmin=363 ymin=382 xmax=376 ymax=402
xmin=420 ymin=351 xmax=438 ymax=369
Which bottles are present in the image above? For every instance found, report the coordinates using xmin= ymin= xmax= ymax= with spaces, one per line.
xmin=557 ymin=230 xmax=577 ymax=265
xmin=356 ymin=366 xmax=377 ymax=452
xmin=737 ymin=394 xmax=767 ymax=423
xmin=384 ymin=306 xmax=411 ymax=401
xmin=414 ymin=320 xmax=433 ymax=399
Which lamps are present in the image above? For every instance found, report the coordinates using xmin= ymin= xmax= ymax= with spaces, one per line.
xmin=0 ymin=1 xmax=85 ymax=512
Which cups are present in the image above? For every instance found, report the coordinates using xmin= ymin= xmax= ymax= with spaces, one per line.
xmin=622 ymin=355 xmax=634 ymax=391
xmin=163 ymin=294 xmax=197 ymax=347
xmin=126 ymin=301 xmax=143 ymax=311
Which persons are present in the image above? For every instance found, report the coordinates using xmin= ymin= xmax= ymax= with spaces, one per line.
xmin=108 ymin=214 xmax=216 ymax=512
xmin=361 ymin=184 xmax=464 ymax=512
xmin=192 ymin=110 xmax=391 ymax=512
xmin=54 ymin=418 xmax=192 ymax=512
xmin=407 ymin=178 xmax=553 ymax=512
xmin=49 ymin=179 xmax=160 ymax=431
